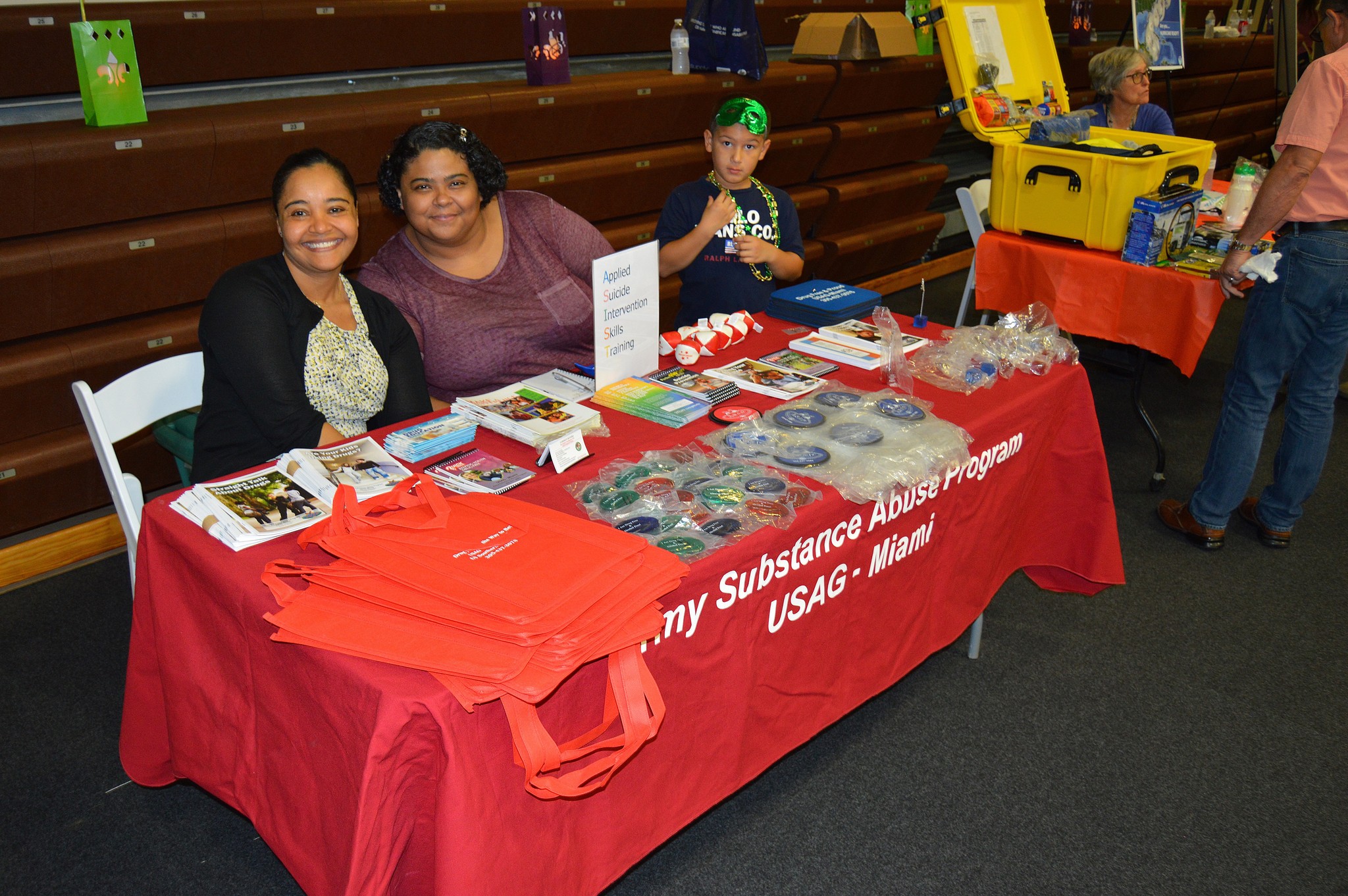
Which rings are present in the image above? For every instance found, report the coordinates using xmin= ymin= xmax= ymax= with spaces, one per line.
xmin=1229 ymin=278 xmax=1234 ymax=283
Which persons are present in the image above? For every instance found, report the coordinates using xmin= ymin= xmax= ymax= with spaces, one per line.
xmin=692 ymin=377 xmax=729 ymax=391
xmin=233 ymin=486 xmax=316 ymax=526
xmin=732 ymin=361 xmax=813 ymax=392
xmin=460 ymin=472 xmax=481 ymax=482
xmin=778 ymin=357 xmax=819 ymax=371
xmin=1076 ymin=46 xmax=1177 ymax=138
xmin=356 ymin=122 xmax=619 ymax=412
xmin=1155 ymin=0 xmax=1348 ymax=548
xmin=483 ymin=396 xmax=569 ymax=424
xmin=652 ymin=93 xmax=804 ymax=331
xmin=330 ymin=459 xmax=389 ymax=484
xmin=850 ymin=327 xmax=881 ymax=344
xmin=189 ymin=148 xmax=434 ymax=486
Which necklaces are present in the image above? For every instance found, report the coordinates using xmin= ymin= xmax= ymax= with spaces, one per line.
xmin=1106 ymin=101 xmax=1137 ymax=130
xmin=709 ymin=170 xmax=780 ymax=281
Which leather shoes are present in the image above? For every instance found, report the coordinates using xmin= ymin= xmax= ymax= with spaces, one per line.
xmin=1156 ymin=498 xmax=1226 ymax=552
xmin=1237 ymin=496 xmax=1292 ymax=548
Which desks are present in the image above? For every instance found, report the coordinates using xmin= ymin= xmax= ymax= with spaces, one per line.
xmin=975 ymin=179 xmax=1277 ymax=487
xmin=118 ymin=313 xmax=1125 ymax=896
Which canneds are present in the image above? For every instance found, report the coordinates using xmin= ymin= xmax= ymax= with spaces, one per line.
xmin=1238 ymin=20 xmax=1248 ymax=36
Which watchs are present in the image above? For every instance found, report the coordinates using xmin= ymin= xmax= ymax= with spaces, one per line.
xmin=1228 ymin=236 xmax=1252 ymax=251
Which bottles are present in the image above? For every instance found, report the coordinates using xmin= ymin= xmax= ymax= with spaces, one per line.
xmin=1246 ymin=10 xmax=1253 ymax=36
xmin=1238 ymin=10 xmax=1244 ymax=21
xmin=670 ymin=19 xmax=690 ymax=75
xmin=1224 ymin=162 xmax=1256 ymax=226
xmin=1229 ymin=10 xmax=1240 ymax=29
xmin=1204 ymin=10 xmax=1215 ymax=39
xmin=1201 ymin=141 xmax=1217 ymax=191
xmin=1090 ymin=28 xmax=1097 ymax=42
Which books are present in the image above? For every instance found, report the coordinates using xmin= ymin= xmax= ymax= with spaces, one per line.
xmin=423 ymin=448 xmax=536 ymax=497
xmin=759 ymin=348 xmax=840 ymax=378
xmin=648 ymin=365 xmax=742 ymax=408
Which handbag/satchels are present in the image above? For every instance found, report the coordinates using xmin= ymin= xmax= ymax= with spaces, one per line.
xmin=258 ymin=474 xmax=691 ymax=806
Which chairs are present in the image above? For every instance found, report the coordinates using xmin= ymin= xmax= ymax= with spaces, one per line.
xmin=71 ymin=352 xmax=204 ymax=603
xmin=955 ymin=179 xmax=1004 ymax=326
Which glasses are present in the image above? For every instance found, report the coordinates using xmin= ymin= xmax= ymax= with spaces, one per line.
xmin=1120 ymin=69 xmax=1152 ymax=85
xmin=1308 ymin=10 xmax=1344 ymax=43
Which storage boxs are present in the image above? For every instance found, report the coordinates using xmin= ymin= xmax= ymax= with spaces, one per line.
xmin=785 ymin=11 xmax=918 ymax=60
xmin=909 ymin=0 xmax=1217 ymax=252
xmin=1121 ymin=185 xmax=1204 ymax=268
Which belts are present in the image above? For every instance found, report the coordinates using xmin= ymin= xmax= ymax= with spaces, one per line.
xmin=1277 ymin=219 xmax=1348 ymax=234
